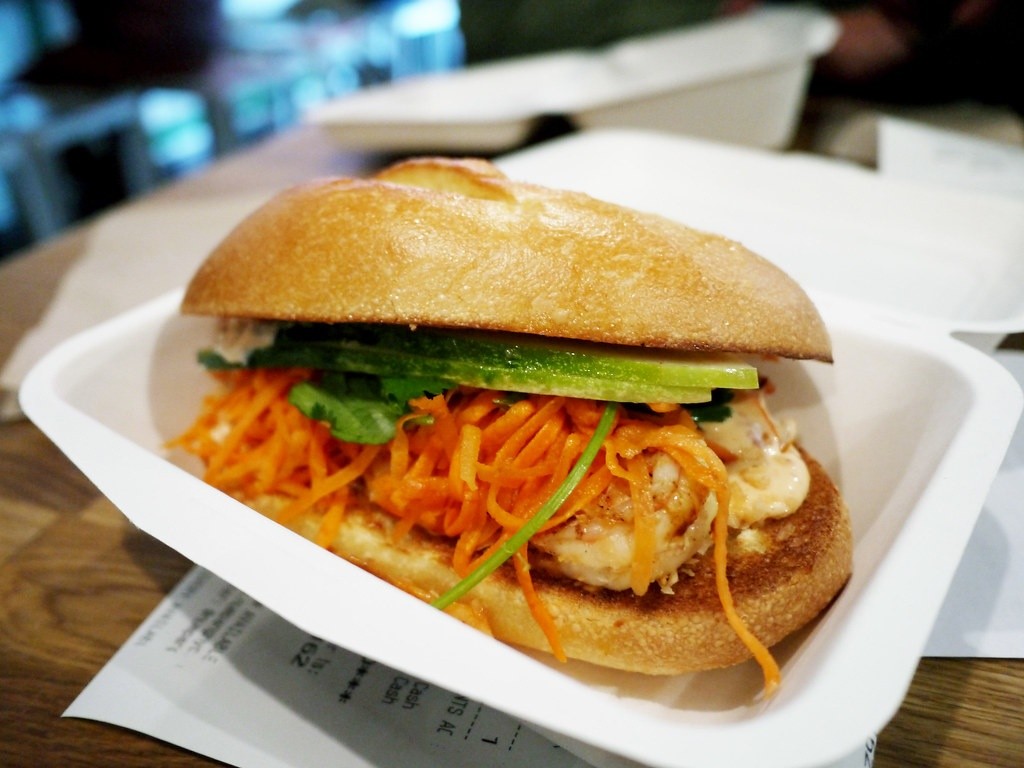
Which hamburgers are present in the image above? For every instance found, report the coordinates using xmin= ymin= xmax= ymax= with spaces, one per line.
xmin=169 ymin=157 xmax=855 ymax=706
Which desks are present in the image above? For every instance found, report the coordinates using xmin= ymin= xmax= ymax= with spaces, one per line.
xmin=0 ymin=133 xmax=1024 ymax=768
